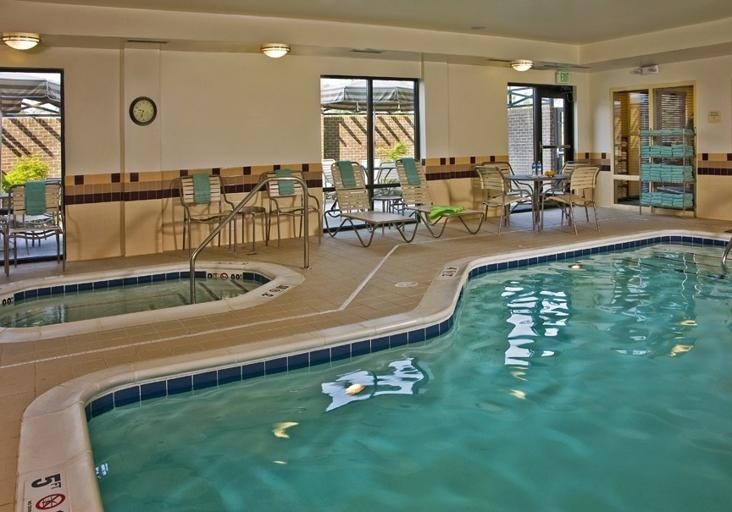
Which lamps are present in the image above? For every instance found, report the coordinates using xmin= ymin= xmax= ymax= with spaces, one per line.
xmin=261 ymin=43 xmax=290 ymax=58
xmin=2 ymin=32 xmax=40 ymax=50
xmin=511 ymin=59 xmax=534 ymax=72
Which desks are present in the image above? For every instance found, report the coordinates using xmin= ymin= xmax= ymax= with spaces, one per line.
xmin=506 ymin=174 xmax=567 ymax=232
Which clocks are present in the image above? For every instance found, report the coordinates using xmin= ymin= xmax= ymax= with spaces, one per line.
xmin=129 ymin=96 xmax=157 ymax=126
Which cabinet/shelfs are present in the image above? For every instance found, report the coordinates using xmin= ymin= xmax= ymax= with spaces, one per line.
xmin=639 ymin=126 xmax=696 ymax=219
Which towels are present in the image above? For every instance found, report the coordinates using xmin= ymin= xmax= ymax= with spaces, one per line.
xmin=640 ymin=129 xmax=693 ymax=208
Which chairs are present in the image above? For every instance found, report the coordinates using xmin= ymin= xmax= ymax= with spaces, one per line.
xmin=540 ymin=161 xmax=600 ymax=235
xmin=0 ymin=178 xmax=64 ymax=276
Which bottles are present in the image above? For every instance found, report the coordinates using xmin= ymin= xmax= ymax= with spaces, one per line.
xmin=536 ymin=161 xmax=541 ymax=176
xmin=531 ymin=161 xmax=536 ymax=176
